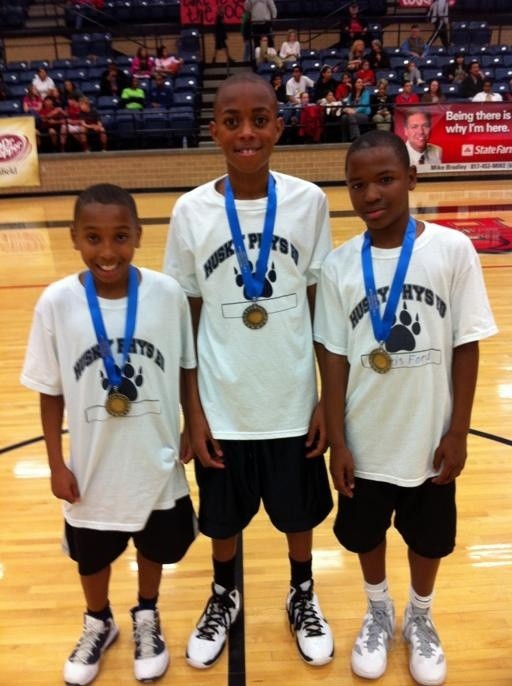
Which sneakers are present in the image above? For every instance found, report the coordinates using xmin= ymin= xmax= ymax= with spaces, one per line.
xmin=63 ymin=606 xmax=118 ymax=686
xmin=403 ymin=601 xmax=446 ymax=686
xmin=351 ymin=599 xmax=395 ymax=680
xmin=185 ymin=583 xmax=240 ymax=669
xmin=130 ymin=606 xmax=168 ymax=681
xmin=287 ymin=577 xmax=334 ymax=666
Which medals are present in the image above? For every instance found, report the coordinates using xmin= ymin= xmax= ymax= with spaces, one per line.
xmin=105 ymin=391 xmax=131 ymax=417
xmin=242 ymin=303 xmax=268 ymax=329
xmin=369 ymin=348 xmax=392 ymax=374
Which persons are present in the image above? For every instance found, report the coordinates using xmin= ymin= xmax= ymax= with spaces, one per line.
xmin=163 ymin=72 xmax=336 ymax=670
xmin=20 ymin=183 xmax=198 ymax=686
xmin=312 ymin=130 xmax=500 ymax=686
xmin=403 ymin=107 xmax=442 ymax=164
xmin=1 ymin=0 xmax=511 ymax=152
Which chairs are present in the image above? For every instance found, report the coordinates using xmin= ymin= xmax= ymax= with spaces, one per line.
xmin=0 ymin=0 xmax=511 ymax=147
